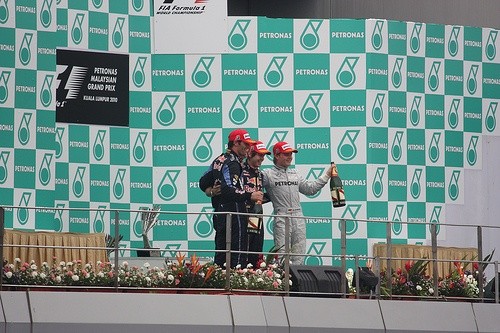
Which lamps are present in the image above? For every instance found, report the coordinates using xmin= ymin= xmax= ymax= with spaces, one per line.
xmin=288 ymin=265 xmax=350 ymax=299
xmin=352 ymin=267 xmax=379 ymax=293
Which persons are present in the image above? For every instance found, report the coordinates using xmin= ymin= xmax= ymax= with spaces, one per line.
xmin=261 ymin=141 xmax=338 ymax=265
xmin=198 ymin=129 xmax=272 ymax=270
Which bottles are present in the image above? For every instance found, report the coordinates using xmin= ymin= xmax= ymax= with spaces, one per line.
xmin=330 ymin=162 xmax=346 ymax=207
xmin=246 ymin=190 xmax=263 ymax=235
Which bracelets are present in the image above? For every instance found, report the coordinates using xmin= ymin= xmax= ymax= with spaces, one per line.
xmin=210 ymin=190 xmax=212 ymax=195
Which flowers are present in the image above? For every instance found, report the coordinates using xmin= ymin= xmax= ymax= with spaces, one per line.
xmin=0 ymin=243 xmax=293 ymax=292
xmin=376 ymin=249 xmax=494 ymax=298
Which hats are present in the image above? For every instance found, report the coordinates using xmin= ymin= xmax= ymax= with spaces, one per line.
xmin=273 ymin=141 xmax=298 ymax=154
xmin=250 ymin=141 xmax=271 ymax=155
xmin=228 ymin=128 xmax=257 ymax=144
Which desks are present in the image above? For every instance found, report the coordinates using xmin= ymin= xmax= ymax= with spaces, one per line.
xmin=0 ymin=229 xmax=107 ymax=273
xmin=373 ymin=244 xmax=478 ymax=278
